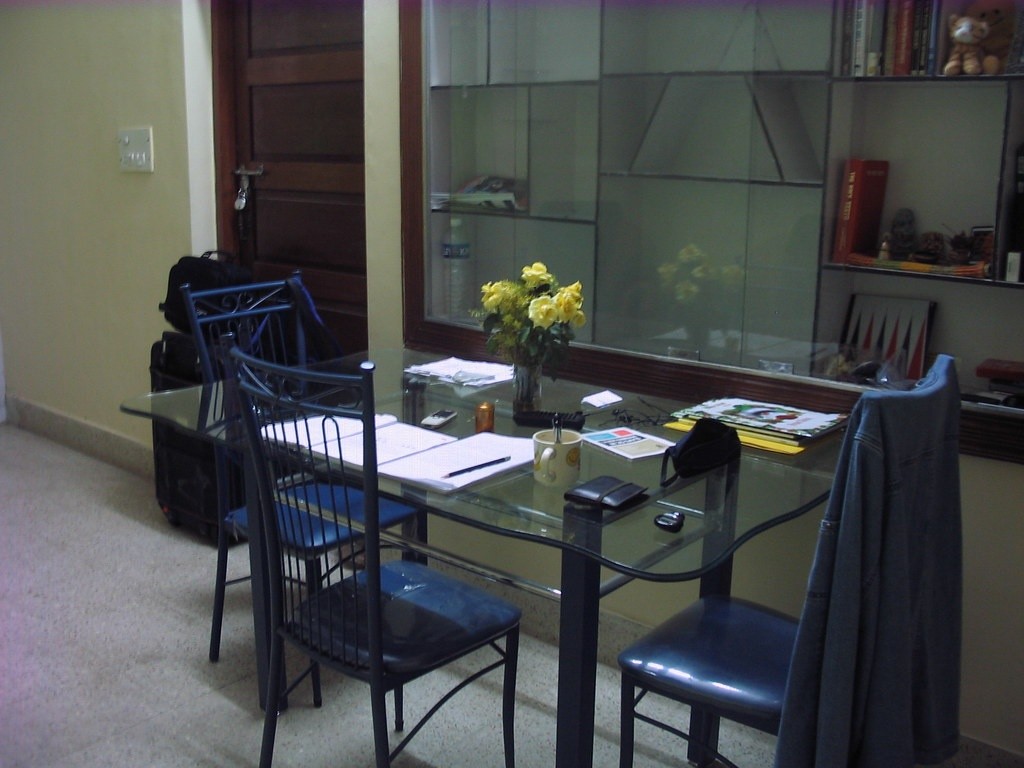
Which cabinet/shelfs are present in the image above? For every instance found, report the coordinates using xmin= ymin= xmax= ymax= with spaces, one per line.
xmin=400 ymin=0 xmax=1024 ymax=468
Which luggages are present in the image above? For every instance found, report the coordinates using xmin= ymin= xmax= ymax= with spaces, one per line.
xmin=149 ymin=331 xmax=251 ymax=547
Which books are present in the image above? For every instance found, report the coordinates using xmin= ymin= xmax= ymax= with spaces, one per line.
xmin=960 ymin=358 xmax=1024 ymax=415
xmin=377 ymin=430 xmax=535 ymax=495
xmin=259 ymin=410 xmax=458 ymax=471
xmin=830 ymin=156 xmax=890 ymax=264
xmin=581 ymin=427 xmax=676 ymax=463
xmin=840 ymin=0 xmax=967 ymax=79
xmin=663 ymin=396 xmax=851 ymax=455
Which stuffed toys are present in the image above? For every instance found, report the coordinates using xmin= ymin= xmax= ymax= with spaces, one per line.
xmin=943 ymin=0 xmax=1017 ymax=76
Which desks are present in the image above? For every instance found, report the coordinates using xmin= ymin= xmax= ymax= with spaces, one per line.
xmin=120 ymin=344 xmax=837 ymax=768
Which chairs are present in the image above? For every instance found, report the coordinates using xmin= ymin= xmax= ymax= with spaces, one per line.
xmin=182 ymin=273 xmax=522 ymax=768
xmin=620 ymin=354 xmax=963 ymax=768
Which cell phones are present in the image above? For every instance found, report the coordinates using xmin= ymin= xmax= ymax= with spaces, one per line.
xmin=420 ymin=409 xmax=458 ymax=430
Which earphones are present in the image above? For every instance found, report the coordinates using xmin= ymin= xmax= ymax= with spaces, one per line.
xmin=613 ymin=408 xmax=623 ymax=414
xmin=619 ymin=416 xmax=626 ymax=422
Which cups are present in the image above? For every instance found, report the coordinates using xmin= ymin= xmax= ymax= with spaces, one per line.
xmin=532 ymin=429 xmax=582 ymax=488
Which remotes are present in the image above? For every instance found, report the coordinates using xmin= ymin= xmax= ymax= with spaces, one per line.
xmin=513 ymin=411 xmax=585 ymax=432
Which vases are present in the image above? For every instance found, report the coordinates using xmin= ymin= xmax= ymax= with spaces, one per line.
xmin=510 ymin=344 xmax=545 ymax=426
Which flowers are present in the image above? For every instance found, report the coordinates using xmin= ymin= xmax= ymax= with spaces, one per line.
xmin=478 ymin=262 xmax=587 ymax=402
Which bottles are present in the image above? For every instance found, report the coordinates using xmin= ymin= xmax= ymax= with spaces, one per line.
xmin=475 ymin=401 xmax=495 ymax=433
xmin=438 ymin=218 xmax=471 ymax=317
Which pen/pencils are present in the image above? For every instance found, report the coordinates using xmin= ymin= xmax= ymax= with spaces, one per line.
xmin=440 ymin=457 xmax=511 ymax=479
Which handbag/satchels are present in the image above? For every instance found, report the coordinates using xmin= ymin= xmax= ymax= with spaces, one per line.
xmin=160 ymin=250 xmax=257 ymax=339
